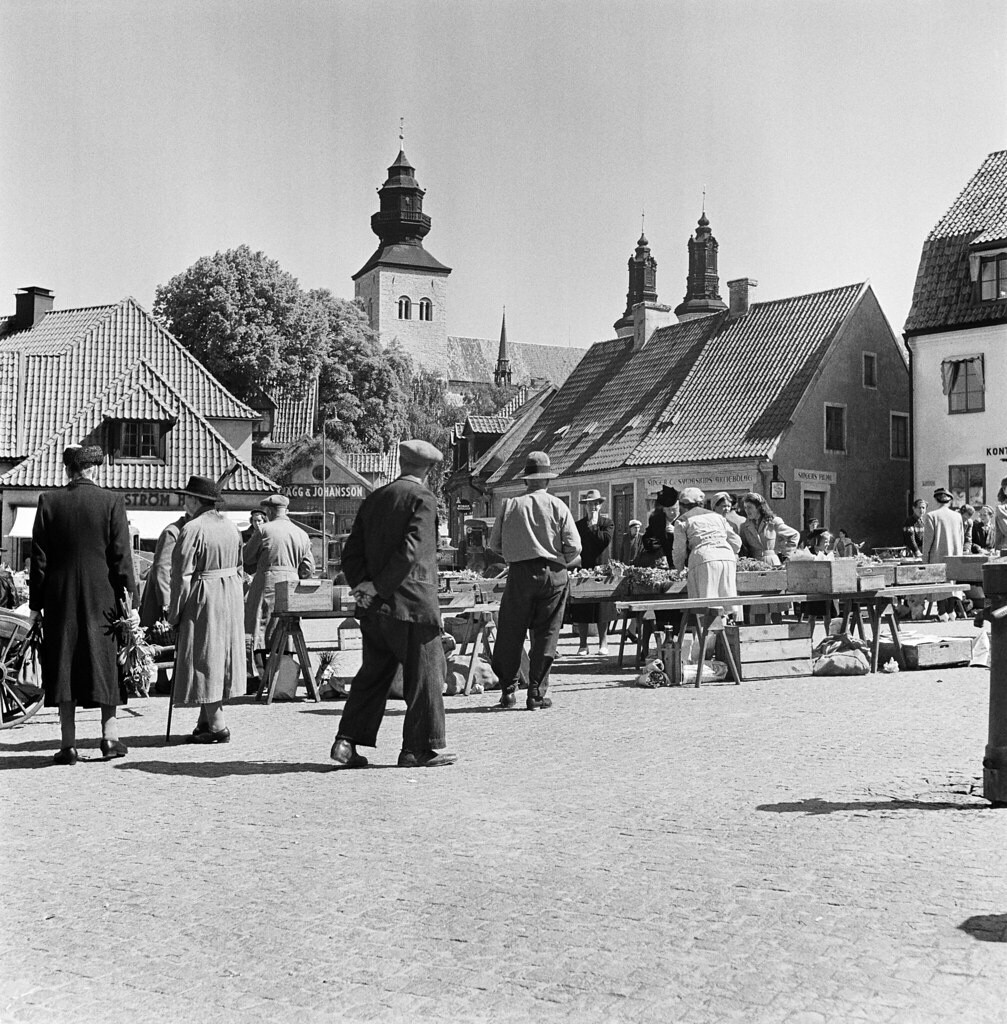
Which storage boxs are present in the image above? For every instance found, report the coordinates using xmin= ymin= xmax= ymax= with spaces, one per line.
xmin=857 ymin=563 xmax=896 ymax=589
xmin=787 ymin=558 xmax=857 ymax=593
xmin=476 ymin=581 xmax=508 ymax=604
xmin=895 ymin=564 xmax=946 ymax=586
xmin=273 ymin=582 xmax=336 ymax=612
xmin=722 ymin=622 xmax=815 ymax=679
xmin=739 ymin=569 xmax=787 ymax=592
xmin=334 ymin=585 xmax=358 ymax=611
xmin=630 ymin=580 xmax=666 ymax=593
xmin=871 ymin=634 xmax=975 ymax=667
xmin=935 ymin=553 xmax=987 ymax=580
xmin=568 ymin=575 xmax=626 ymax=597
xmin=436 ymin=589 xmax=475 ymax=608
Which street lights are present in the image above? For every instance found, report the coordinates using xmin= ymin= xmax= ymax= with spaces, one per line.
xmin=319 ymin=406 xmax=342 ymax=580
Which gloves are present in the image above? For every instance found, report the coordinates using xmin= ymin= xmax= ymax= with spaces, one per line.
xmin=646 ymin=538 xmax=662 ymax=549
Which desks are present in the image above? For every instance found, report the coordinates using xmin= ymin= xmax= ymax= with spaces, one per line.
xmin=259 ymin=581 xmax=986 ymax=715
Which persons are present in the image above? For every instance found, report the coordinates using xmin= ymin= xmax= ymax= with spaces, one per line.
xmin=902 ymin=477 xmax=1007 ymax=621
xmin=242 ymin=495 xmax=316 ymax=696
xmin=620 ymin=483 xmax=853 ymax=628
xmin=142 ymin=507 xmax=193 ymax=695
xmin=169 ymin=476 xmax=246 ymax=740
xmin=30 ymin=445 xmax=141 ymax=761
xmin=671 ymin=486 xmax=744 ymax=627
xmin=489 ymin=452 xmax=582 ymax=709
xmin=330 ymin=440 xmax=458 ymax=766
xmin=573 ymin=488 xmax=615 ymax=655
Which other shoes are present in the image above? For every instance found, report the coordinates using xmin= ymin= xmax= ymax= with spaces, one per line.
xmin=577 ymin=646 xmax=589 ymax=656
xmin=598 ymin=647 xmax=609 ymax=655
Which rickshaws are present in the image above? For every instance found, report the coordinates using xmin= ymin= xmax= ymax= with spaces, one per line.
xmin=0 ymin=602 xmax=176 ymax=729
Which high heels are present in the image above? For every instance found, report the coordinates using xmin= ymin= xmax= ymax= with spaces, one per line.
xmin=947 ymin=610 xmax=957 ymax=621
xmin=932 ymin=612 xmax=949 ymax=622
xmin=623 ymin=629 xmax=638 ymax=643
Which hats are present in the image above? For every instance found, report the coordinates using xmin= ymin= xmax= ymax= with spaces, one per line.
xmin=806 ymin=528 xmax=828 ymax=540
xmin=260 ymin=494 xmax=290 ymax=507
xmin=933 ymin=487 xmax=956 ymax=502
xmin=679 ymin=488 xmax=705 ymax=504
xmin=578 ymin=489 xmax=606 ymax=504
xmin=518 ymin=452 xmax=559 ymax=480
xmin=629 ymin=519 xmax=643 ymax=527
xmin=174 ymin=476 xmax=225 ymax=502
xmin=710 ymin=492 xmax=729 ymax=510
xmin=658 ymin=485 xmax=678 ymax=507
xmin=399 ymin=440 xmax=444 ymax=466
xmin=251 ymin=509 xmax=266 ymax=516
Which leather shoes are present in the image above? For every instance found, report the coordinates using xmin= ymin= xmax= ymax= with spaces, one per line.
xmin=100 ymin=738 xmax=128 ymax=757
xmin=526 ymin=696 xmax=552 ymax=710
xmin=398 ymin=750 xmax=458 ymax=767
xmin=500 ymin=693 xmax=516 ymax=708
xmin=330 ymin=738 xmax=368 ymax=766
xmin=192 ymin=726 xmax=231 ymax=743
xmin=54 ymin=747 xmax=78 ymax=765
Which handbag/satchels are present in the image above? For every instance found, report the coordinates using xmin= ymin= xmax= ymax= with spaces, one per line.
xmin=17 ymin=620 xmax=46 ymax=695
xmin=635 ymin=543 xmax=669 ymax=570
